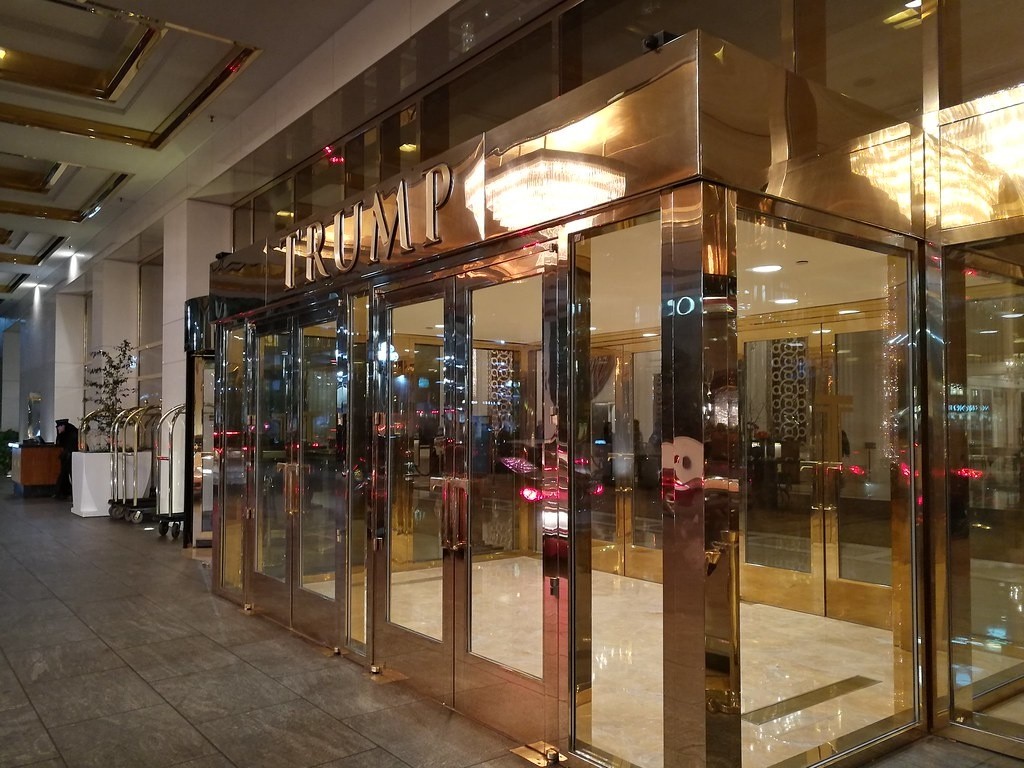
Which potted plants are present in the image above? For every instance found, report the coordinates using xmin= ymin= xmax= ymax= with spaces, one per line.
xmin=71 ymin=339 xmax=153 ymax=518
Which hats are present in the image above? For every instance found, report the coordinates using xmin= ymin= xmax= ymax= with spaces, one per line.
xmin=56 ymin=419 xmax=69 ymax=427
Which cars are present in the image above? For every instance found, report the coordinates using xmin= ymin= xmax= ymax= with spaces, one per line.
xmin=540 ymin=444 xmax=592 ymax=475
xmin=213 ymin=432 xmax=247 ymax=495
xmin=471 ymin=444 xmax=605 ymax=513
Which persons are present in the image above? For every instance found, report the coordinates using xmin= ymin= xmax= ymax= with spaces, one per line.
xmin=604 ymin=422 xmax=613 ymax=444
xmin=55 ymin=419 xmax=88 ymax=498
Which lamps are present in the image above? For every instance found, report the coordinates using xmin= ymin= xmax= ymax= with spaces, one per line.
xmin=852 ymin=82 xmax=1024 ymax=229
xmin=484 ymin=150 xmax=624 ymax=274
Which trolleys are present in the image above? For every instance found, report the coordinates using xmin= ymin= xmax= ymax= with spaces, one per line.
xmin=108 ymin=406 xmax=158 ymax=524
xmin=152 ymin=402 xmax=185 ymax=539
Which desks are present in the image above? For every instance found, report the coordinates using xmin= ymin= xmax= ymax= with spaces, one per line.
xmin=8 ymin=441 xmax=64 ymax=496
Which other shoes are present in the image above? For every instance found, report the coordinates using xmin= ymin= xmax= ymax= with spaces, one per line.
xmin=52 ymin=494 xmax=59 ymax=498
